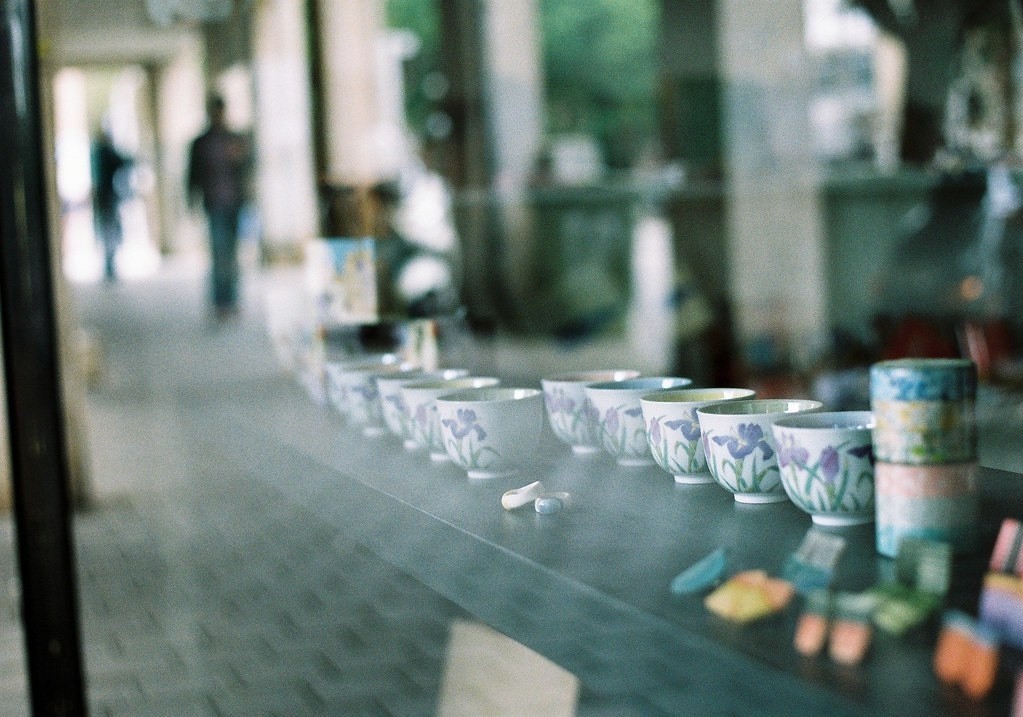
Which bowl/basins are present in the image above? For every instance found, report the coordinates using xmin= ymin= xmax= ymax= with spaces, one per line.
xmin=541 ymin=368 xmax=875 ymax=525
xmin=327 ymin=354 xmax=541 ymax=480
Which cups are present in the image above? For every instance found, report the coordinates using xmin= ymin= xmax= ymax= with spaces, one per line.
xmin=870 ymin=357 xmax=977 ymax=558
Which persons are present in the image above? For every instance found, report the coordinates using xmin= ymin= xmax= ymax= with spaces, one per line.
xmin=359 ymin=179 xmax=417 ymax=378
xmin=186 ymin=95 xmax=254 ymax=322
xmin=91 ymin=134 xmax=134 ymax=284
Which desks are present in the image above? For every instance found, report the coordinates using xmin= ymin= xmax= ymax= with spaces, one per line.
xmin=228 ymin=394 xmax=1023 ymax=717
